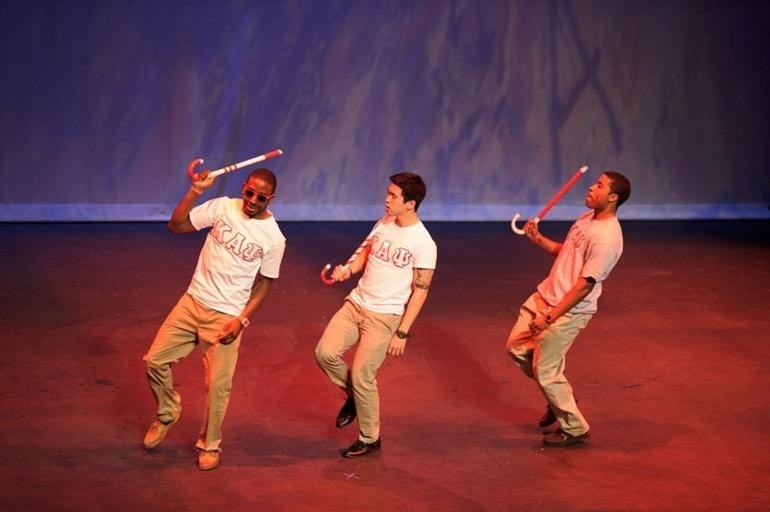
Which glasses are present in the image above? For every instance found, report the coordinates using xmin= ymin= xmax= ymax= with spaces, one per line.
xmin=243 ymin=183 xmax=274 ymax=202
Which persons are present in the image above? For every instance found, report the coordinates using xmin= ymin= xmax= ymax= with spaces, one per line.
xmin=142 ymin=168 xmax=287 ymax=470
xmin=314 ymin=172 xmax=438 ymax=459
xmin=505 ymin=171 xmax=631 ymax=447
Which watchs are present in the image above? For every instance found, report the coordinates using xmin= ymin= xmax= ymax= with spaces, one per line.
xmin=236 ymin=315 xmax=250 ymax=328
xmin=544 ymin=312 xmax=557 ymax=325
xmin=395 ymin=328 xmax=409 ymax=339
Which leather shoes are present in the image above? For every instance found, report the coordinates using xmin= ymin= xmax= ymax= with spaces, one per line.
xmin=144 ymin=413 xmax=180 ymax=447
xmin=342 ymin=438 xmax=382 ymax=457
xmin=198 ymin=445 xmax=220 ymax=470
xmin=539 ymin=396 xmax=579 ymax=427
xmin=336 ymin=399 xmax=357 ymax=427
xmin=542 ymin=426 xmax=590 ymax=446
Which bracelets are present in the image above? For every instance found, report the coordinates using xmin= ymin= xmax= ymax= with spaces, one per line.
xmin=189 ymin=185 xmax=203 ymax=195
xmin=347 ymin=265 xmax=353 ymax=278
xmin=531 ymin=231 xmax=544 ymax=245
